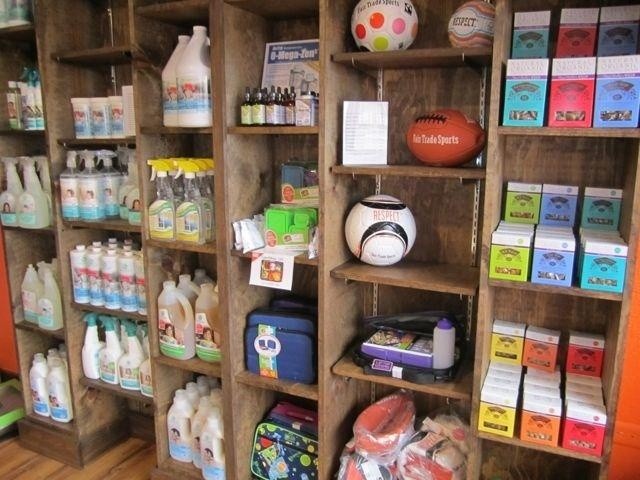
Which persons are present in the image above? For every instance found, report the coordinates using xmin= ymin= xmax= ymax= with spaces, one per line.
xmin=202 ymin=447 xmax=217 ymax=467
xmin=63 ymin=189 xmax=78 ymax=206
xmin=8 ymin=100 xmax=19 ymax=120
xmin=129 ymin=199 xmax=141 ymax=212
xmin=2 ymin=202 xmax=11 ymax=214
xmin=33 ymin=390 xmax=40 ymax=402
xmin=51 ymin=395 xmax=61 ymax=407
xmin=144 ymin=374 xmax=153 ymax=386
xmin=82 ymin=190 xmax=97 ymax=206
xmin=122 ymin=195 xmax=128 ymax=206
xmin=169 ymin=428 xmax=182 ymax=446
xmin=105 ymin=188 xmax=115 ymax=203
xmin=159 ymin=323 xmax=179 ymax=345
xmin=125 ymin=368 xmax=133 ymax=380
xmin=192 ymin=436 xmax=201 ymax=453
xmin=198 ymin=327 xmax=218 ymax=349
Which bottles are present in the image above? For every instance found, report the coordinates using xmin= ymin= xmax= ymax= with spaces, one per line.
xmin=68 ymin=237 xmax=147 ymax=317
xmin=4 ymin=80 xmax=21 ymax=129
xmin=240 ymin=87 xmax=319 ymax=127
xmin=432 ymin=317 xmax=457 ymax=370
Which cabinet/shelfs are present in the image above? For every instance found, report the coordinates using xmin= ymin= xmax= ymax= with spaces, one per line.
xmin=0 ymin=0 xmax=640 ymax=480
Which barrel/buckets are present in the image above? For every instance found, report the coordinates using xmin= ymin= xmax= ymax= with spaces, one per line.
xmin=163 ymin=375 xmax=227 ymax=480
xmin=156 ymin=266 xmax=223 ymax=363
xmin=28 ymin=341 xmax=74 ymax=424
xmin=161 ymin=25 xmax=213 ymax=128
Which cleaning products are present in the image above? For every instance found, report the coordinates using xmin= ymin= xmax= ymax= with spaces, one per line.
xmin=21 ymin=256 xmax=64 ymax=331
xmin=80 ymin=310 xmax=154 ymax=398
xmin=0 ymin=154 xmax=54 ymax=230
xmin=146 ymin=155 xmax=217 ymax=245
xmin=5 ymin=65 xmax=48 ymax=132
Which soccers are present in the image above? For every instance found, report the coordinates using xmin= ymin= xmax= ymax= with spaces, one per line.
xmin=345 ymin=195 xmax=416 ymax=266
xmin=448 ymin=0 xmax=495 ymax=48
xmin=351 ymin=0 xmax=418 ymax=51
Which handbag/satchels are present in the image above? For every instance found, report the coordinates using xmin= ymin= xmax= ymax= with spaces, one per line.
xmin=250 ymin=401 xmax=319 ymax=479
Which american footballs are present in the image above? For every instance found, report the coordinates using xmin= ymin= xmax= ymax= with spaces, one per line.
xmin=406 ymin=110 xmax=487 ymax=166
